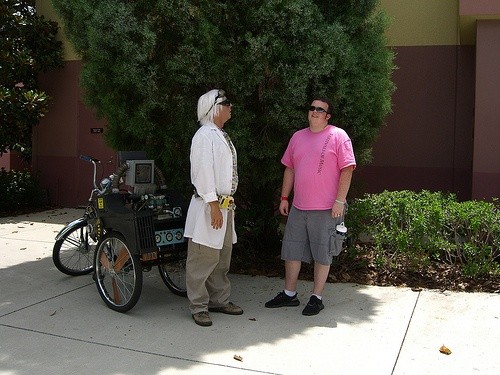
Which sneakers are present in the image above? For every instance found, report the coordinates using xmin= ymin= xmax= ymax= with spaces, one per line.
xmin=265 ymin=289 xmax=300 ymax=308
xmin=302 ymin=295 xmax=324 ymax=315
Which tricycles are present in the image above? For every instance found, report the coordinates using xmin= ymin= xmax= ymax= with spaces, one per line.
xmin=51 ymin=153 xmax=208 ymax=313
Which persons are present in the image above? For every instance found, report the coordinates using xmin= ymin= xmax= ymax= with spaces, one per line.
xmin=184 ymin=89 xmax=244 ymax=327
xmin=265 ymin=98 xmax=356 ymax=316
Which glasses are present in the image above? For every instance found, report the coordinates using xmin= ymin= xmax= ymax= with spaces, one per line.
xmin=217 ymin=99 xmax=231 ymax=106
xmin=309 ymin=105 xmax=328 ymax=114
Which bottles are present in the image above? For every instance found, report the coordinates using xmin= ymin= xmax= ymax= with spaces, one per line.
xmin=335 ymin=221 xmax=347 ymax=237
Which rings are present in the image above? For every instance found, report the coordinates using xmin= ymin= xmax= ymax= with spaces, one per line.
xmin=337 ymin=214 xmax=340 ymax=217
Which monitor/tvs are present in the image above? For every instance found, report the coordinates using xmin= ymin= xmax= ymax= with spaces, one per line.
xmin=125 ymin=160 xmax=154 ymax=188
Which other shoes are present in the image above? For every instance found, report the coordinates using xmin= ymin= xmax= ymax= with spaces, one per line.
xmin=191 ymin=311 xmax=212 ymax=326
xmin=208 ymin=302 xmax=244 ymax=315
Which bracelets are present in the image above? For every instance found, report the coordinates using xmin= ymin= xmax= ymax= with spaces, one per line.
xmin=281 ymin=196 xmax=288 ymax=200
xmin=335 ymin=200 xmax=345 ymax=205
